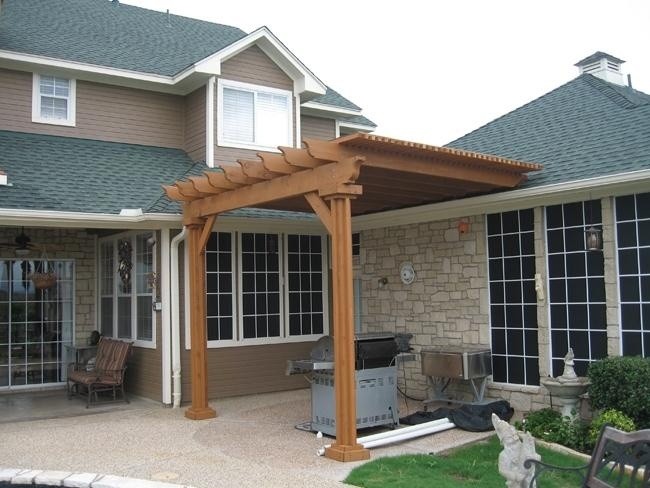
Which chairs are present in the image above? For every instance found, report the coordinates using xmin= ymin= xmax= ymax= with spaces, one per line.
xmin=523 ymin=424 xmax=650 ymax=488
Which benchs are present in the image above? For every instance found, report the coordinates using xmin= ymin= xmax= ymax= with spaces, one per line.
xmin=67 ymin=338 xmax=136 ymax=407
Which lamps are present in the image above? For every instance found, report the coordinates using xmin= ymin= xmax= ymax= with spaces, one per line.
xmin=583 ymin=227 xmax=604 ymax=253
xmin=15 ymin=248 xmax=31 ymax=255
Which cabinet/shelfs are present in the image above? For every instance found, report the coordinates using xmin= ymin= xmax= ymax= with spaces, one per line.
xmin=65 ymin=344 xmax=98 ymax=393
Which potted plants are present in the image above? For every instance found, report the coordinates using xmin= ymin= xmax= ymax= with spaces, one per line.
xmin=29 ymin=270 xmax=56 ymax=289
